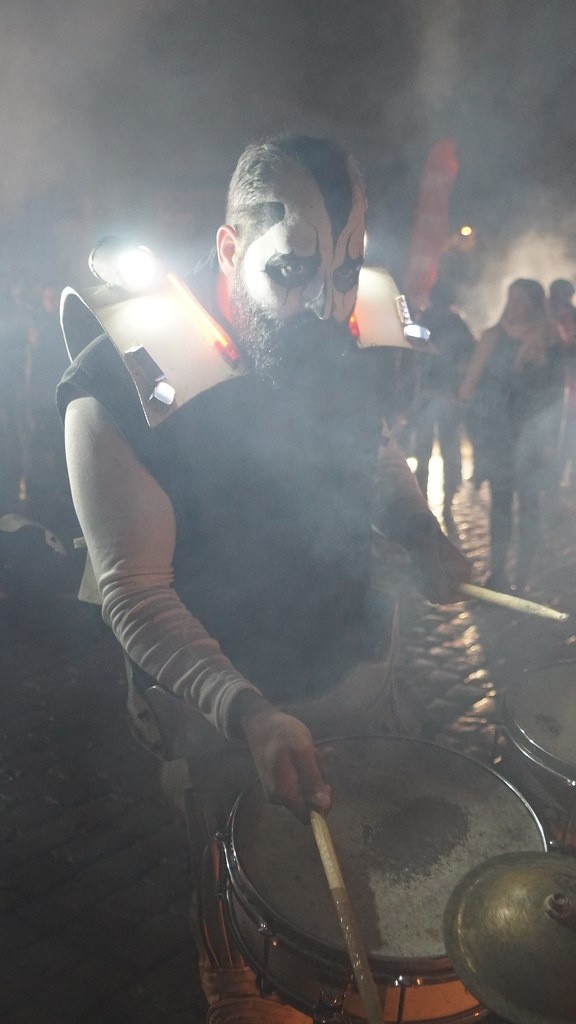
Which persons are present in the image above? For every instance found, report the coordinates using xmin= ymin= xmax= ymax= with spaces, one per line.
xmin=407 ymin=281 xmax=477 ymax=527
xmin=25 ymin=283 xmax=69 ymax=429
xmin=52 ymin=134 xmax=485 ymax=902
xmin=546 ymin=277 xmax=575 ymax=333
xmin=455 ymin=278 xmax=568 ymax=596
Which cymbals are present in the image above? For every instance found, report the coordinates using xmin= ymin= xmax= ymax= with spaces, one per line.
xmin=443 ymin=852 xmax=576 ymax=1024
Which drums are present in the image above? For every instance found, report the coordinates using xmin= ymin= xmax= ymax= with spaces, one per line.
xmin=501 ymin=655 xmax=575 ymax=789
xmin=218 ymin=730 xmax=548 ymax=1023
